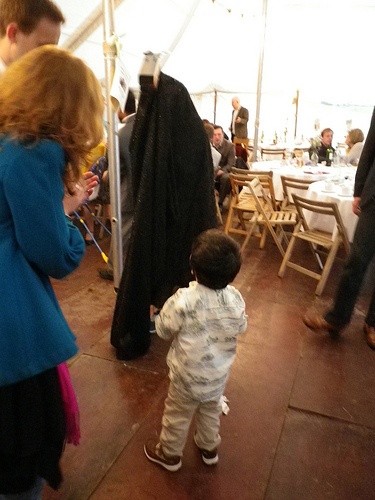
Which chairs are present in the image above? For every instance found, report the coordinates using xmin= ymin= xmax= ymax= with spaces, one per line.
xmin=214 ymin=137 xmax=351 ymax=295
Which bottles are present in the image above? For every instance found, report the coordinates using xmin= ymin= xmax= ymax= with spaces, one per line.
xmin=326 ymin=143 xmax=333 ymax=166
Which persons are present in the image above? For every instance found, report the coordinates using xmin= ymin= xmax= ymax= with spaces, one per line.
xmin=302 ymin=106 xmax=375 ymax=349
xmin=0 ymin=0 xmax=364 ymax=472
xmin=0 ymin=45 xmax=103 ymax=500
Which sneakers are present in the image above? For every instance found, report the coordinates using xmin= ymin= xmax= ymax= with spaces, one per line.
xmin=201 ymin=447 xmax=219 ymax=465
xmin=144 ymin=439 xmax=182 ymax=472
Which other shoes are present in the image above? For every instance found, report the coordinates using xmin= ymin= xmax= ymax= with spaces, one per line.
xmin=363 ymin=323 xmax=375 ymax=349
xmin=85 ymin=238 xmax=93 ymax=246
xmin=98 ymin=268 xmax=113 ymax=280
xmin=301 ymin=316 xmax=348 ymax=338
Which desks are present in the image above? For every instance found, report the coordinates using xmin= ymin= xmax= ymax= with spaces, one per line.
xmin=257 ymin=142 xmax=311 ymax=161
xmin=243 ymin=160 xmax=358 ymax=204
xmin=300 ymin=175 xmax=359 ymax=243
